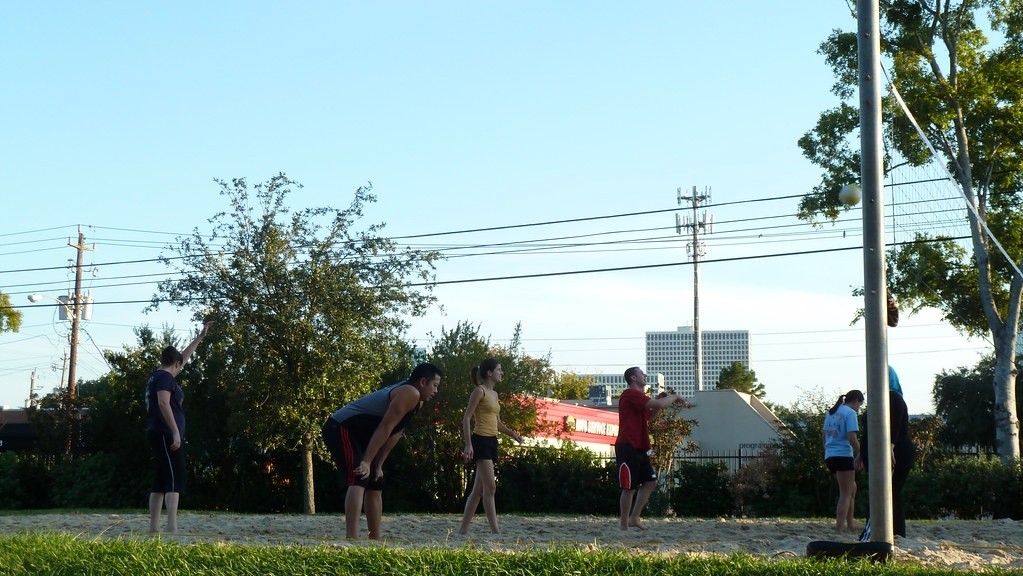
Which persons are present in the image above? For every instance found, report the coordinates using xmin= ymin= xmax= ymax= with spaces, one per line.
xmin=459 ymin=357 xmax=526 ymax=534
xmin=144 ymin=321 xmax=215 ymax=532
xmin=821 ymin=390 xmax=863 ymax=534
xmin=858 ymin=363 xmax=917 ymax=541
xmin=321 ymin=363 xmax=444 ymax=538
xmin=615 ymin=366 xmax=686 ymax=532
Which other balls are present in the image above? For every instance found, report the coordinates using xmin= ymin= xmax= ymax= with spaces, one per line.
xmin=839 ymin=185 xmax=861 ymax=205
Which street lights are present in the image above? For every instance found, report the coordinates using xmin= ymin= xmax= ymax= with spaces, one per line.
xmin=28 ymin=293 xmax=82 ymax=453
